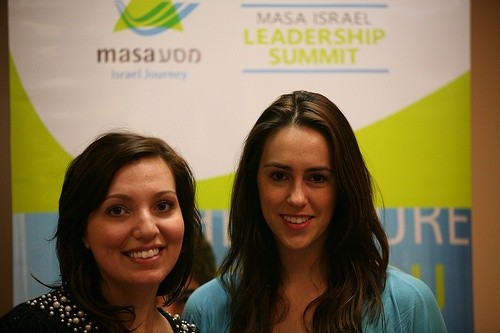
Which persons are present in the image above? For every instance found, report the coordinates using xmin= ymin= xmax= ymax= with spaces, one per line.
xmin=177 ymin=90 xmax=448 ymax=333
xmin=1 ymin=127 xmax=203 ymax=333
xmin=155 ymin=226 xmax=217 ymax=319
